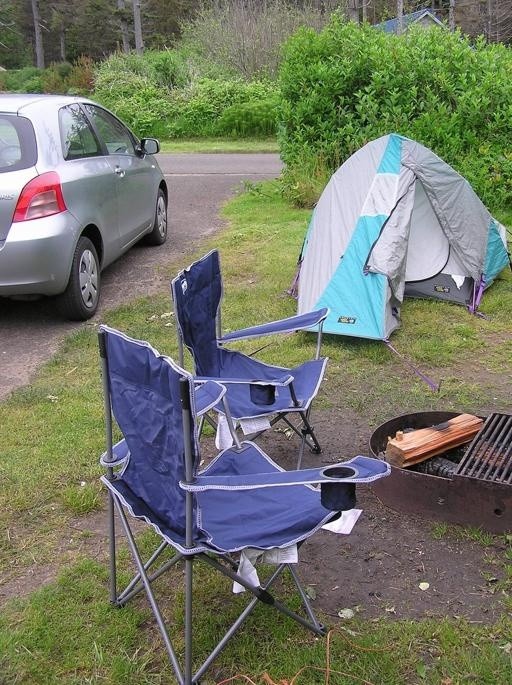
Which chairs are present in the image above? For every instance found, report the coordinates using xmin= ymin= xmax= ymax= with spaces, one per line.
xmin=168 ymin=248 xmax=331 ymax=468
xmin=97 ymin=325 xmax=393 ymax=685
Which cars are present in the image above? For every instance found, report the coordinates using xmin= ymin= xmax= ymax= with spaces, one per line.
xmin=0 ymin=95 xmax=167 ymax=319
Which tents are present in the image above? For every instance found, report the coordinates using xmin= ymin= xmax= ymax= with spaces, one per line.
xmin=286 ymin=132 xmax=512 ymax=341
